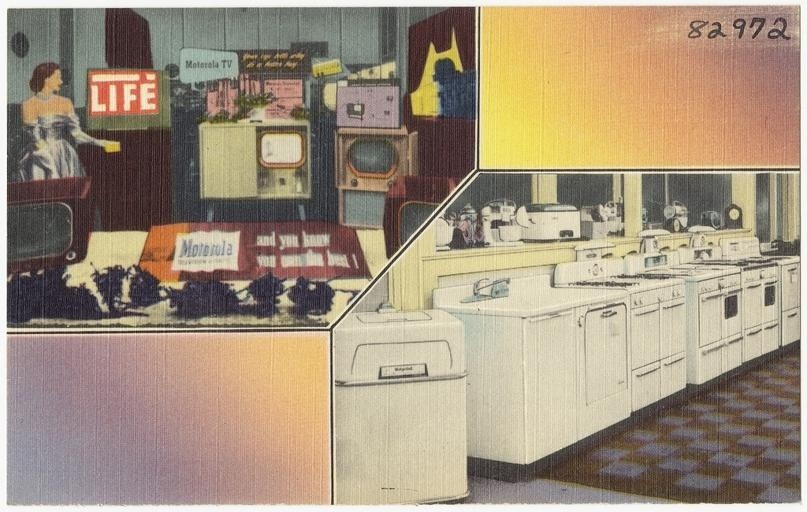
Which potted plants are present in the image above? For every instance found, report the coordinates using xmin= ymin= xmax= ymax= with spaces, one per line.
xmin=233 ymin=90 xmax=280 ymax=124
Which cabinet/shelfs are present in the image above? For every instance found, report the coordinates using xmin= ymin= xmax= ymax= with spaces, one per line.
xmin=198 ymin=118 xmax=314 ymax=223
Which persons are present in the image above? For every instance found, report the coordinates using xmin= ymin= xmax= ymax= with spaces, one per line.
xmin=17 ymin=61 xmax=110 ymax=232
